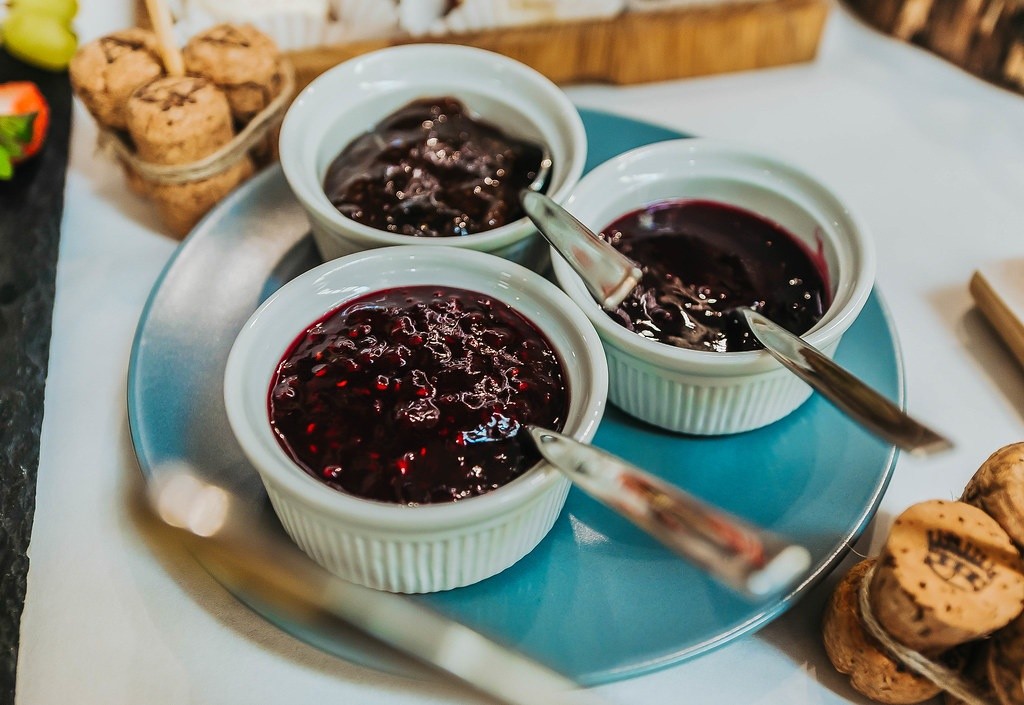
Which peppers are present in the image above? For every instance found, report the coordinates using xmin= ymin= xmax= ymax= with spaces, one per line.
xmin=0 ymin=0 xmax=81 ymax=177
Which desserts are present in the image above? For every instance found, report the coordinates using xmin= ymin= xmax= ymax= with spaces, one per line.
xmin=68 ymin=23 xmax=290 ymax=239
xmin=821 ymin=441 xmax=1024 ymax=705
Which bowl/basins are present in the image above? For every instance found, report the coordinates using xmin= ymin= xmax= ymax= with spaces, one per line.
xmin=223 ymin=245 xmax=612 ymax=592
xmin=551 ymin=137 xmax=878 ymax=435
xmin=278 ymin=42 xmax=587 ymax=277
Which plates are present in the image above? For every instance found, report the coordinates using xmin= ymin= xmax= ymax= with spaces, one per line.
xmin=128 ymin=107 xmax=907 ymax=685
